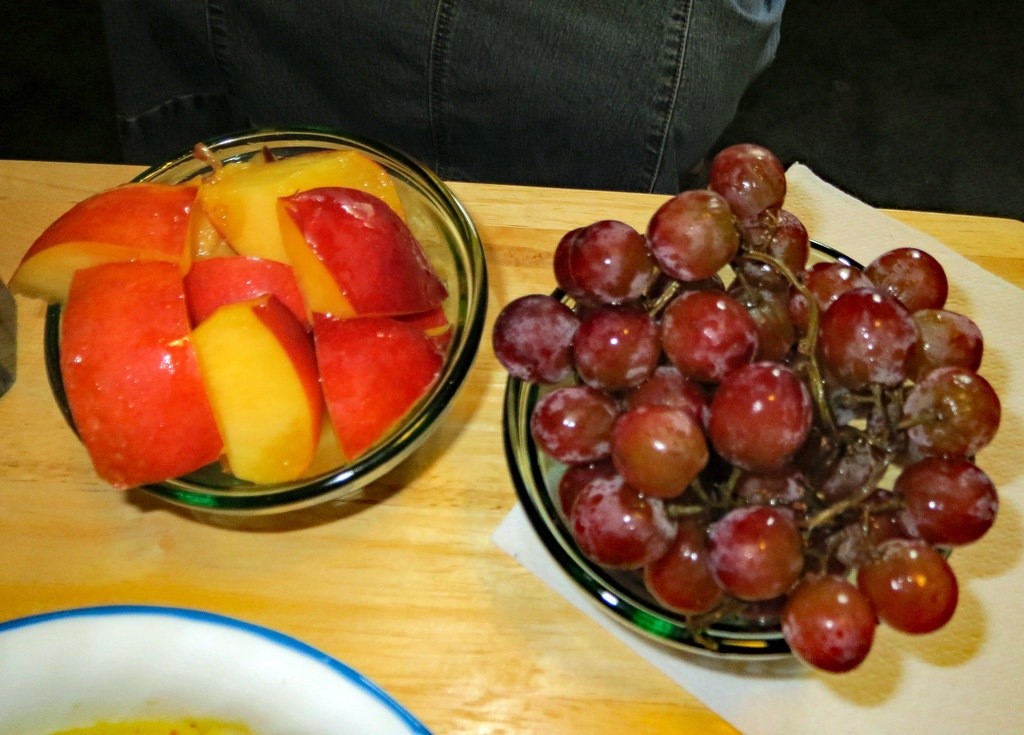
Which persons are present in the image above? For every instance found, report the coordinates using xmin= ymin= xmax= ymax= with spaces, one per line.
xmin=105 ymin=1 xmax=785 ymax=196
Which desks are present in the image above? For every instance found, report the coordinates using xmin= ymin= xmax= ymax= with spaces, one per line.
xmin=0 ymin=159 xmax=1024 ymax=735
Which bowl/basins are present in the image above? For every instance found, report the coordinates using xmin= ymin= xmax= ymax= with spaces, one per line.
xmin=41 ymin=123 xmax=484 ymax=513
xmin=500 ymin=232 xmax=913 ymax=656
xmin=0 ymin=606 xmax=438 ymax=735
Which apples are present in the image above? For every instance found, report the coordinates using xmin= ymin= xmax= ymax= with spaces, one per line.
xmin=5 ymin=149 xmax=454 ymax=493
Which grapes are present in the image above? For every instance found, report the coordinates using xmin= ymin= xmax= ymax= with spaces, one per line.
xmin=489 ymin=142 xmax=1000 ymax=673
